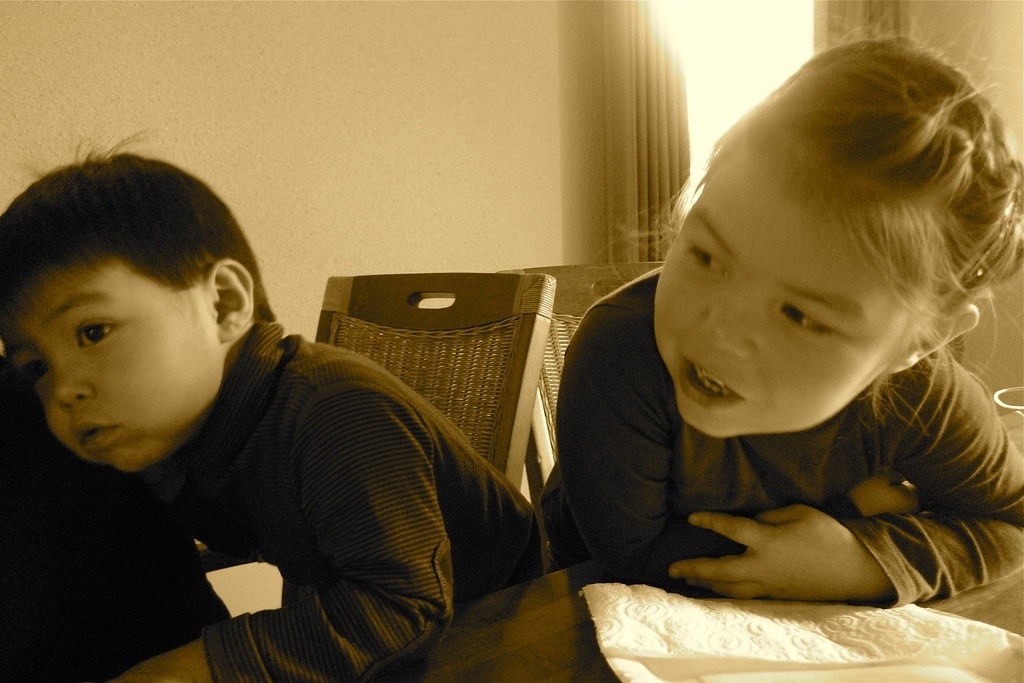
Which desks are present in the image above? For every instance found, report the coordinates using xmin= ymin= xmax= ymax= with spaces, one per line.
xmin=382 ymin=560 xmax=1024 ymax=683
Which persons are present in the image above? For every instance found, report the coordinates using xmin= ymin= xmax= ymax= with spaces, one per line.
xmin=541 ymin=35 xmax=1024 ymax=609
xmin=0 ymin=153 xmax=547 ymax=683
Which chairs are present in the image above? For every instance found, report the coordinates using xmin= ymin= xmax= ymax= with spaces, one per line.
xmin=315 ymin=271 xmax=559 ymax=491
xmin=522 ymin=260 xmax=668 ymax=502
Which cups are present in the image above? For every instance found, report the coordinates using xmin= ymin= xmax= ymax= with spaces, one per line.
xmin=994 ymin=386 xmax=1024 ymax=454
